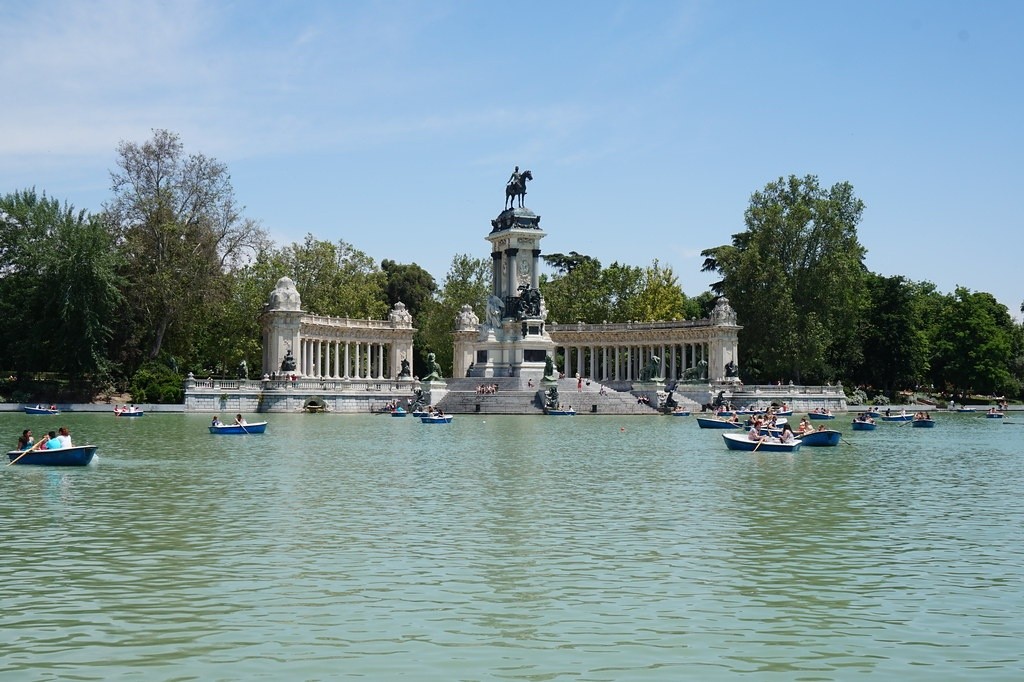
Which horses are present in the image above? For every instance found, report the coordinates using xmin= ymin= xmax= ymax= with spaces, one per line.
xmin=505 ymin=171 xmax=533 ymax=211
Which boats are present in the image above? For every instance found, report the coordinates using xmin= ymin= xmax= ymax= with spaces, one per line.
xmin=795 ymin=430 xmax=843 ymax=447
xmin=858 ymin=412 xmax=881 ymax=419
xmin=548 ymin=409 xmax=576 ymax=416
xmin=412 ymin=411 xmax=439 ymax=417
xmin=912 ymin=419 xmax=935 ymax=428
xmin=881 ymin=414 xmax=914 ymax=421
xmin=696 ymin=416 xmax=744 ymax=429
xmin=851 ymin=421 xmax=877 ymax=431
xmin=208 ymin=421 xmax=268 ymax=434
xmin=670 ymin=411 xmax=690 ymax=416
xmin=420 ymin=414 xmax=454 ymax=423
xmin=391 ymin=411 xmax=407 ymax=417
xmin=7 ymin=444 xmax=99 ymax=466
xmin=986 ymin=413 xmax=1005 ymax=419
xmin=113 ymin=409 xmax=144 ymax=417
xmin=722 ymin=433 xmax=803 ymax=453
xmin=715 ymin=408 xmax=804 ymax=438
xmin=370 ymin=409 xmax=409 ymax=414
xmin=24 ymin=406 xmax=60 ymax=415
xmin=956 ymin=408 xmax=976 ymax=412
xmin=807 ymin=412 xmax=836 ymax=420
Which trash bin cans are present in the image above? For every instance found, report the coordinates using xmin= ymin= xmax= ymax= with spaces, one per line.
xmin=475 ymin=404 xmax=480 ymax=412
xmin=592 ymin=404 xmax=597 ymax=412
xmin=295 ymin=381 xmax=299 ymax=387
xmin=467 ymin=370 xmax=470 ymax=376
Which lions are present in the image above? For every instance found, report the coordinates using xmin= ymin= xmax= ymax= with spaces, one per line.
xmin=427 ymin=353 xmax=442 ymax=377
xmin=680 ymin=360 xmax=708 ymax=380
xmin=639 ymin=356 xmax=662 ymax=380
xmin=544 ymin=354 xmax=554 ymax=377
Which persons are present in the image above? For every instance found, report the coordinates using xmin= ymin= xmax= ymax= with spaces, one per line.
xmin=861 ymin=406 xmax=930 ymax=424
xmin=384 ymin=402 xmax=403 ymax=411
xmin=676 ymin=406 xmax=682 ymax=411
xmin=638 ymin=394 xmax=650 ymax=404
xmin=715 ymin=403 xmax=830 ymax=444
xmin=560 ymin=405 xmax=573 ymax=411
xmin=428 ymin=406 xmax=444 ymax=417
xmin=212 ymin=416 xmax=223 ymax=426
xmin=991 ymin=400 xmax=1007 ymax=413
xmin=578 ymin=376 xmax=582 ymax=389
xmin=476 ymin=383 xmax=497 ymax=394
xmin=50 ymin=404 xmax=56 ymax=410
xmin=507 ymin=165 xmax=521 ymax=186
xmin=517 ymin=282 xmax=537 ymax=300
xmin=17 ymin=427 xmax=72 ymax=450
xmin=508 ymin=364 xmax=513 ymax=376
xmin=286 ymin=374 xmax=297 ymax=388
xmin=114 ymin=404 xmax=138 ymax=412
xmin=320 ymin=377 xmax=324 ymax=387
xmin=236 ymin=414 xmax=247 ymax=425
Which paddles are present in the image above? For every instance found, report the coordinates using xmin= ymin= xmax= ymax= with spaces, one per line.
xmin=234 ymin=417 xmax=249 ymax=434
xmin=753 ymin=437 xmax=765 ymax=453
xmin=48 ymin=410 xmax=55 ymax=415
xmin=901 ymin=412 xmax=907 ymax=421
xmin=720 ymin=416 xmax=741 ymax=428
xmin=5 ymin=438 xmax=46 ymax=466
xmin=443 ymin=416 xmax=448 ymax=424
xmin=794 ymin=429 xmax=815 ymax=439
xmin=840 ymin=437 xmax=851 ymax=445
xmin=900 ymin=418 xmax=916 ymax=427
